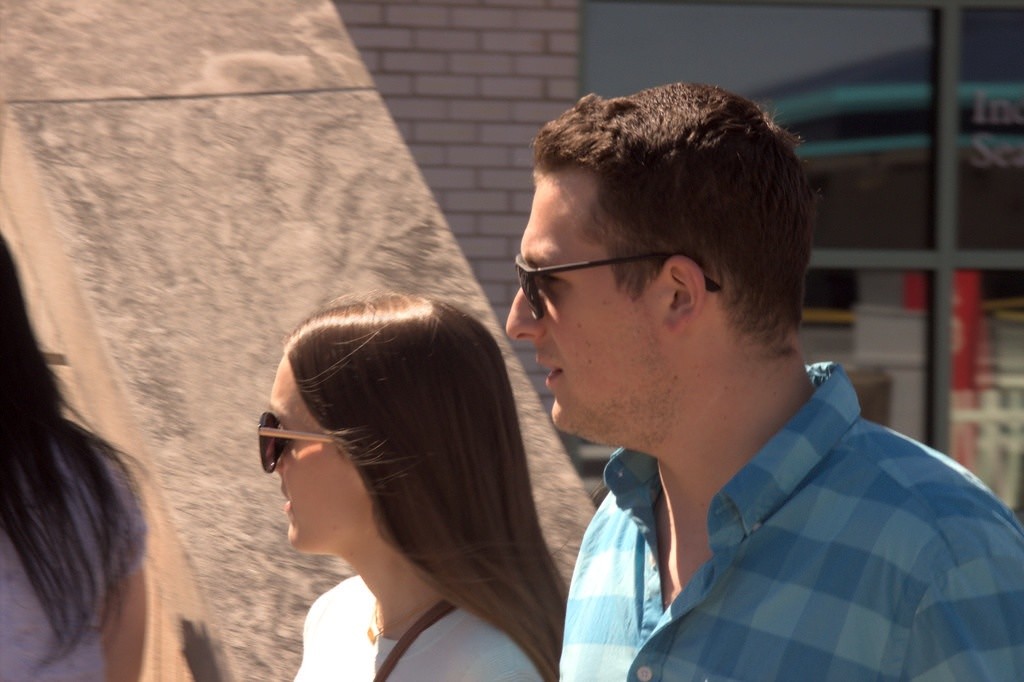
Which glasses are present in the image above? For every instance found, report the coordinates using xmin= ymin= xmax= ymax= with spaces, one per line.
xmin=257 ymin=412 xmax=358 ymax=474
xmin=515 ymin=251 xmax=722 ymax=322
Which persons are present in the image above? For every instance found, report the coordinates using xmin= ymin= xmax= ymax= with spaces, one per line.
xmin=257 ymin=293 xmax=569 ymax=682
xmin=506 ymin=82 xmax=1024 ymax=682
xmin=0 ymin=233 xmax=147 ymax=682
